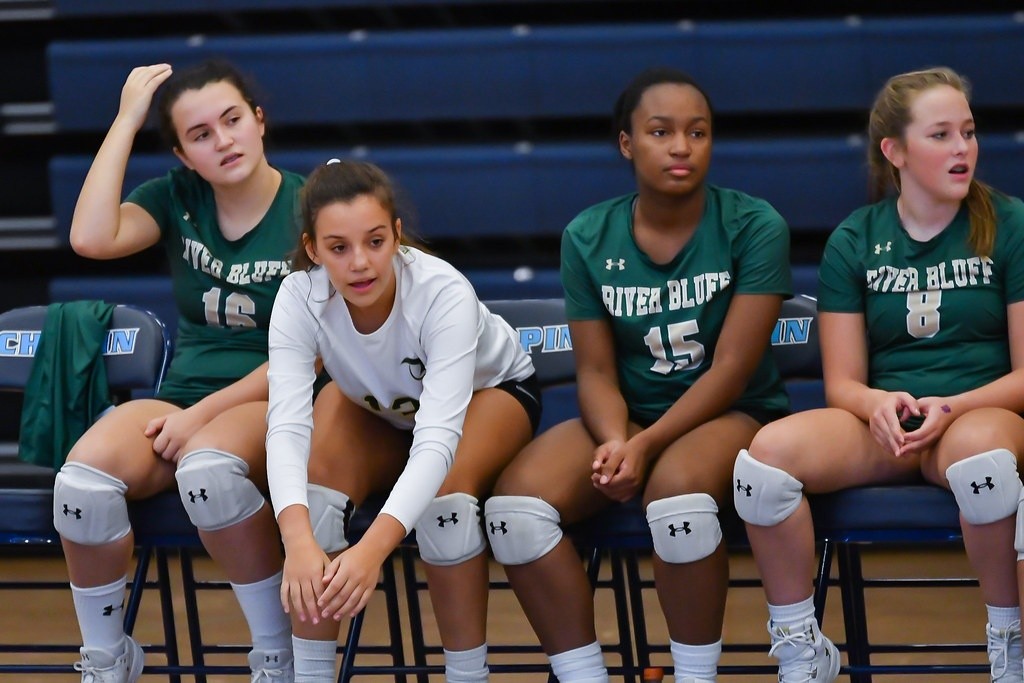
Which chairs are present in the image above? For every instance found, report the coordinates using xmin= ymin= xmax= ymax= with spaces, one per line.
xmin=0 ymin=289 xmax=1024 ymax=683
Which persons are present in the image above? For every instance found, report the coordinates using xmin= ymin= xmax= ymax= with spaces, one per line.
xmin=730 ymin=65 xmax=1024 ymax=683
xmin=483 ymin=68 xmax=795 ymax=683
xmin=265 ymin=157 xmax=542 ymax=683
xmin=52 ymin=58 xmax=323 ymax=683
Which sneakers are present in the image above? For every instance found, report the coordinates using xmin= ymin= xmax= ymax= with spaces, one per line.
xmin=74 ymin=632 xmax=144 ymax=683
xmin=767 ymin=617 xmax=841 ymax=683
xmin=247 ymin=645 xmax=295 ymax=683
xmin=985 ymin=620 xmax=1024 ymax=683
